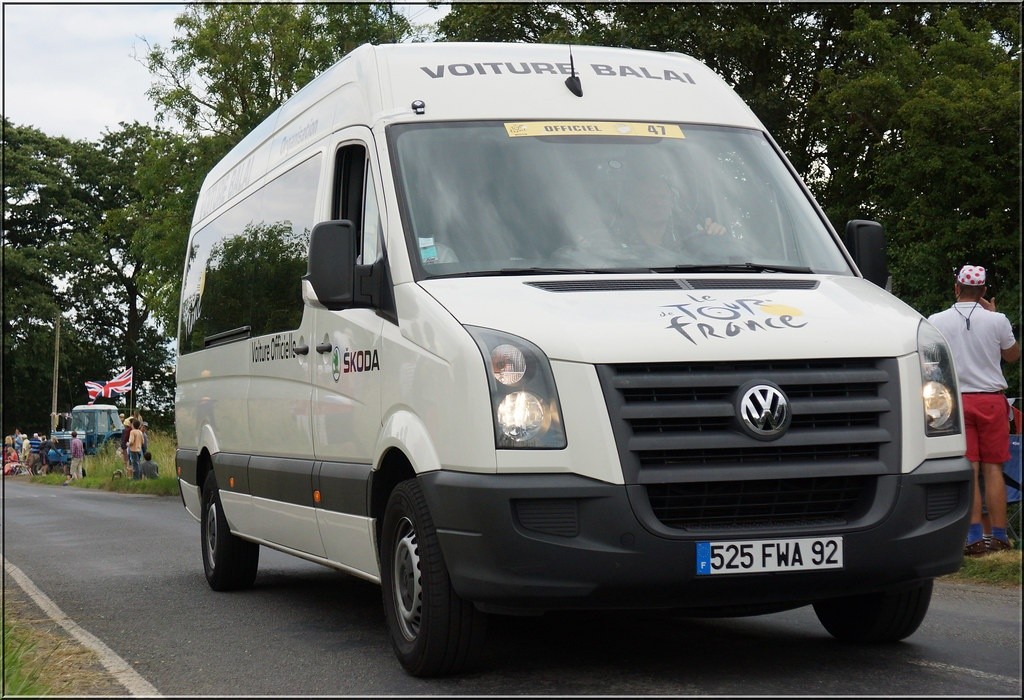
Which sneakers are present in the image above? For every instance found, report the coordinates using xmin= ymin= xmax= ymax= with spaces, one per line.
xmin=990 ymin=537 xmax=1011 ymax=550
xmin=964 ymin=538 xmax=986 ymax=556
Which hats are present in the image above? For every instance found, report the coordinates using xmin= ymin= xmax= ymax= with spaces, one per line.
xmin=957 ymin=265 xmax=986 ymax=286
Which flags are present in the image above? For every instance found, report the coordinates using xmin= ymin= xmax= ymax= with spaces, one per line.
xmin=85 ymin=368 xmax=132 ymax=405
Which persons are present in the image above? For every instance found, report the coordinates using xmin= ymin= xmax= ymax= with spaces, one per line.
xmin=138 ymin=452 xmax=159 ymax=480
xmin=134 ymin=411 xmax=143 ymax=421
xmin=620 ymin=168 xmax=727 ymax=264
xmin=38 ymin=437 xmax=63 ymax=477
xmin=126 ymin=420 xmax=144 ymax=481
xmin=140 ymin=422 xmax=148 ymax=452
xmin=121 ymin=419 xmax=132 ymax=475
xmin=30 ymin=433 xmax=41 ymax=445
xmin=21 ymin=434 xmax=31 ymax=460
xmin=927 ymin=265 xmax=1020 ymax=558
xmin=4 ymin=436 xmax=17 ymax=462
xmin=12 ymin=427 xmax=23 ymax=456
xmin=70 ymin=431 xmax=84 ymax=481
xmin=41 ymin=435 xmax=46 ymax=442
xmin=120 ymin=413 xmax=125 ymax=428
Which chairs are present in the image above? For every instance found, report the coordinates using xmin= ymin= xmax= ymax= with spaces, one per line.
xmin=978 ymin=434 xmax=1022 ymax=541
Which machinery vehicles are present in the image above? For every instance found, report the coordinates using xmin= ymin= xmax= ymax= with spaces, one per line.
xmin=31 ymin=404 xmax=125 ymax=476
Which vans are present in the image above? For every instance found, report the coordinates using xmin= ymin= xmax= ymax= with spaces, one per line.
xmin=171 ymin=39 xmax=975 ymax=682
